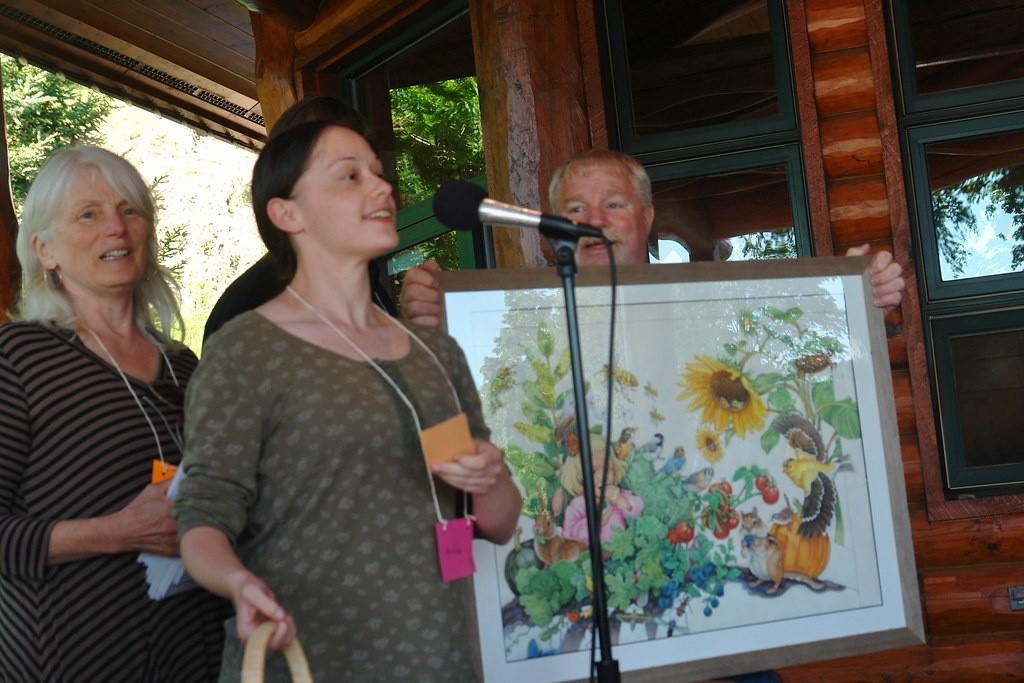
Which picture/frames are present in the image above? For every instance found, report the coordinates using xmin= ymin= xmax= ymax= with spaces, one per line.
xmin=434 ymin=254 xmax=928 ymax=683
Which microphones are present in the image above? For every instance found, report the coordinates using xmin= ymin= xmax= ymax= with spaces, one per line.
xmin=433 ymin=179 xmax=602 ymax=239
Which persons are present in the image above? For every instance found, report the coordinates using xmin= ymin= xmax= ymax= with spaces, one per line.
xmin=549 ymin=151 xmax=906 ymax=319
xmin=172 ymin=97 xmax=521 ymax=682
xmin=0 ymin=146 xmax=236 ymax=682
